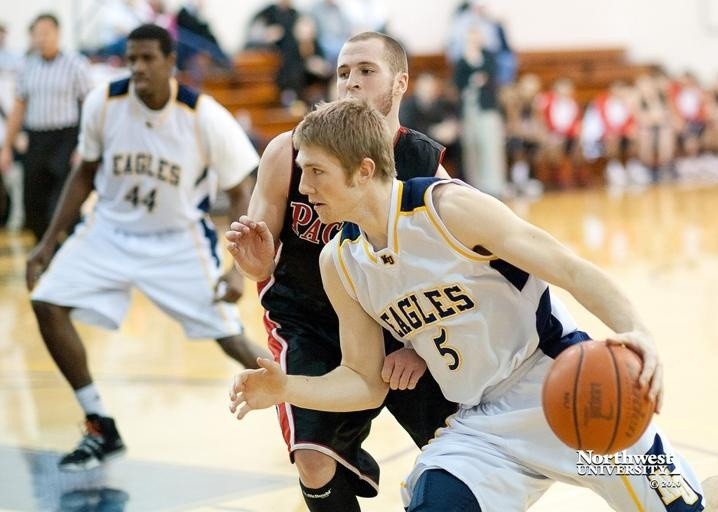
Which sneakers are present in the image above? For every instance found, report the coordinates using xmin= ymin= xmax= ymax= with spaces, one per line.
xmin=56 ymin=413 xmax=126 ymax=473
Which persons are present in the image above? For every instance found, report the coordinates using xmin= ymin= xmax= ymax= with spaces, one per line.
xmin=24 ymin=22 xmax=274 ymax=473
xmin=0 ymin=9 xmax=95 ymax=252
xmin=223 ymin=30 xmax=459 ymax=509
xmin=224 ymin=95 xmax=717 ymax=510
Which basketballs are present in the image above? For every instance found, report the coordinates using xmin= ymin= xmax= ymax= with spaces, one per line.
xmin=542 ymin=340 xmax=657 ymax=455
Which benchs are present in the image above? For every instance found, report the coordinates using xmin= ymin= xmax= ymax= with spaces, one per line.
xmin=174 ymin=35 xmax=716 ymax=192
